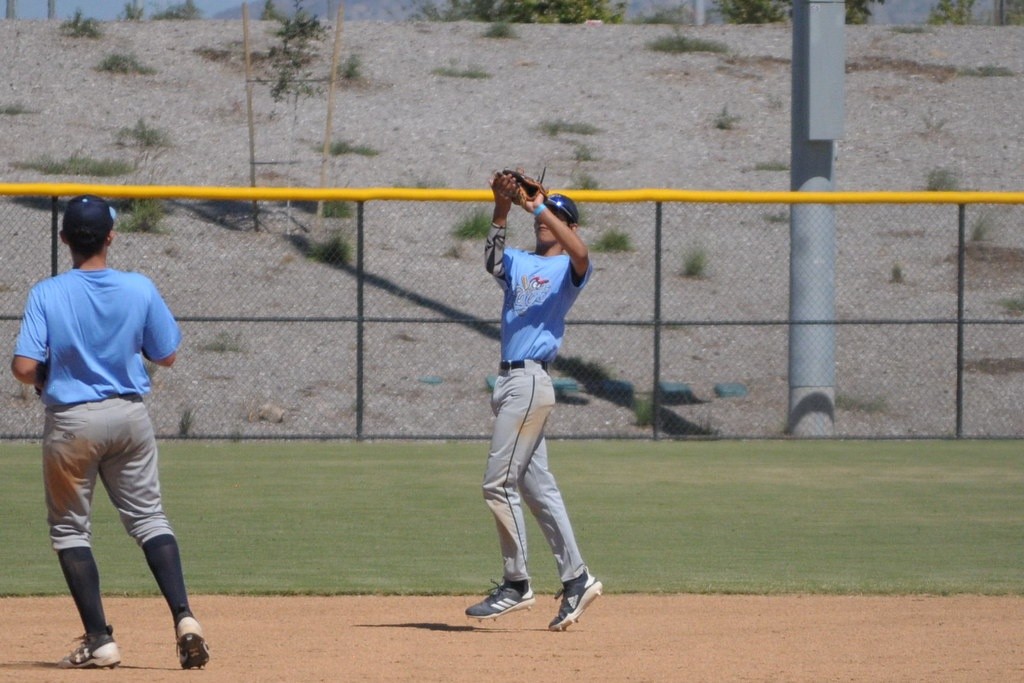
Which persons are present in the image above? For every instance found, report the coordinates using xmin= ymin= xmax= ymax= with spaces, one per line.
xmin=10 ymin=194 xmax=211 ymax=670
xmin=464 ymin=167 xmax=603 ymax=632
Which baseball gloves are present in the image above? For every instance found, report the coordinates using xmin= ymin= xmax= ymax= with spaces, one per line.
xmin=491 ymin=166 xmax=550 ymax=206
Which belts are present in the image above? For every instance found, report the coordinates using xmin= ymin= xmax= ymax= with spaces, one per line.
xmin=499 ymin=360 xmax=548 ymax=374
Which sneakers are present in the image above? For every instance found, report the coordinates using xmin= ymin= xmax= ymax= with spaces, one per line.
xmin=465 ymin=575 xmax=536 ymax=623
xmin=175 ymin=607 xmax=210 ymax=670
xmin=549 ymin=569 xmax=603 ymax=632
xmin=56 ymin=624 xmax=122 ymax=668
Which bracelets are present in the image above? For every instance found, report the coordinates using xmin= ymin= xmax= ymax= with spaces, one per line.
xmin=533 ymin=203 xmax=546 ymax=216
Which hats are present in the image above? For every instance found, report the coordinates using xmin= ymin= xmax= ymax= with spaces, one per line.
xmin=545 ymin=192 xmax=579 ymax=223
xmin=64 ymin=194 xmax=117 ymax=234
